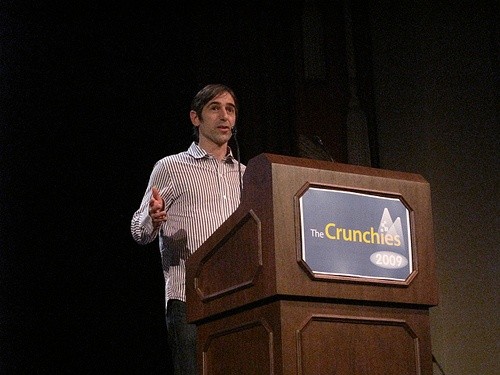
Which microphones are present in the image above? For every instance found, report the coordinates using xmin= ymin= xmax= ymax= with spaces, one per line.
xmin=231 ymin=126 xmax=242 ymax=201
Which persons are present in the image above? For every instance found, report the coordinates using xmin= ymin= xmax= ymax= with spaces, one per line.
xmin=131 ymin=83 xmax=248 ymax=375
